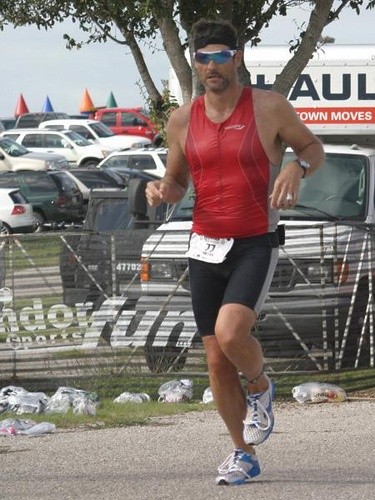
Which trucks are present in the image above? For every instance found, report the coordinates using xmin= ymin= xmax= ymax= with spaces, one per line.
xmin=133 ymin=41 xmax=375 ymax=376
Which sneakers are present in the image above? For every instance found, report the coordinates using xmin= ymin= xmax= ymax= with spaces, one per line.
xmin=214 ymin=448 xmax=262 ymax=486
xmin=243 ymin=374 xmax=274 ymax=445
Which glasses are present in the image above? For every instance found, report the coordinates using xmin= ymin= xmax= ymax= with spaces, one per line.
xmin=192 ymin=48 xmax=238 ymax=65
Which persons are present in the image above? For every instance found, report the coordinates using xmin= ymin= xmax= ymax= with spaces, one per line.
xmin=143 ymin=17 xmax=324 ymax=486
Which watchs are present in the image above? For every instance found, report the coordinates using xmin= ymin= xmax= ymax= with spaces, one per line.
xmin=294 ymin=158 xmax=311 ymax=178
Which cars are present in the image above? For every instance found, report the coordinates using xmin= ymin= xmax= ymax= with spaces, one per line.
xmin=0 ymin=104 xmax=170 ymax=326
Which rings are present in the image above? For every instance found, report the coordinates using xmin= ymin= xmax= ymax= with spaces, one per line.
xmin=286 ymin=194 xmax=293 ymax=200
